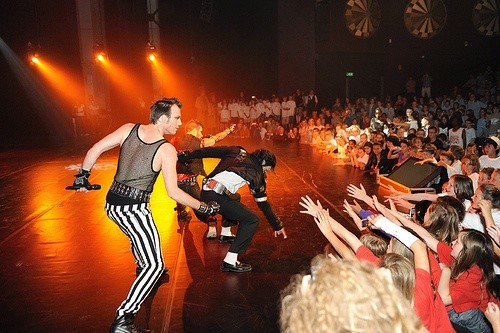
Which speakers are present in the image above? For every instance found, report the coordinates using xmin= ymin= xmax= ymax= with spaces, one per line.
xmin=387 ymin=157 xmax=450 ymax=194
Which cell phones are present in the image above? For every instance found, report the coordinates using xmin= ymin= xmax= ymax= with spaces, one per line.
xmin=410 ymin=207 xmax=415 ymax=218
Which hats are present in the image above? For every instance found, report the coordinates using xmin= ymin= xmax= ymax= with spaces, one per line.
xmin=488 ymin=136 xmax=500 ymax=147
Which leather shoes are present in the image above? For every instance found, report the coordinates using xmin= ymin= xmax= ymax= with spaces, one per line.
xmin=218 ymin=234 xmax=236 ymax=244
xmin=109 ymin=313 xmax=137 ymax=333
xmin=136 ymin=266 xmax=168 ymax=276
xmin=220 ymin=261 xmax=252 ymax=272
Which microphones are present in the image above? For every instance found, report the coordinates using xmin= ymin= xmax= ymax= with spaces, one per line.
xmin=66 ymin=184 xmax=101 ymax=190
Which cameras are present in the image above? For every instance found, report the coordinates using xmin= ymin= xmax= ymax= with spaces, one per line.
xmin=361 ymin=219 xmax=372 ymax=228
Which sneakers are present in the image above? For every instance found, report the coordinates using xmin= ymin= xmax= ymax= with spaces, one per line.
xmin=207 ymin=227 xmax=217 ymax=239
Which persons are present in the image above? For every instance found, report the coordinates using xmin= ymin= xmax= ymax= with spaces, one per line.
xmin=177 ymin=145 xmax=287 ymax=272
xmin=280 ymin=183 xmax=500 ymax=333
xmin=73 ymin=97 xmax=220 ymax=333
xmin=72 ymin=94 xmax=101 ymax=136
xmin=195 ymin=87 xmax=318 ymax=141
xmin=311 ymin=95 xmax=500 ymax=235
xmin=170 ymin=119 xmax=236 ymax=240
